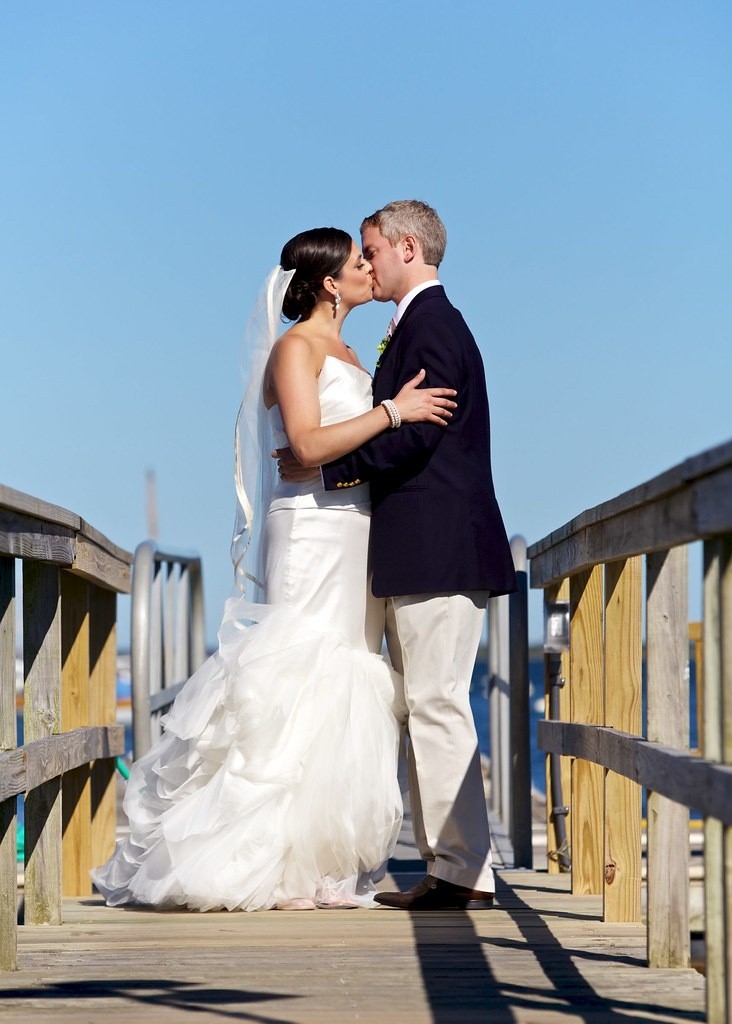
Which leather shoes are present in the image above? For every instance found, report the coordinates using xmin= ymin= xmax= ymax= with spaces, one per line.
xmin=372 ymin=873 xmax=495 ymax=910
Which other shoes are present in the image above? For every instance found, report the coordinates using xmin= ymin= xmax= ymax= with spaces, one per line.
xmin=270 ymin=898 xmax=316 ymax=910
xmin=315 ymin=889 xmax=359 ymax=909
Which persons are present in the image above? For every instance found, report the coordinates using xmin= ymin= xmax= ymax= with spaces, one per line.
xmin=98 ymin=228 xmax=457 ymax=914
xmin=274 ymin=199 xmax=515 ymax=910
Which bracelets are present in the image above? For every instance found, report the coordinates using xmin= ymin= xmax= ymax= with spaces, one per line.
xmin=381 ymin=399 xmax=401 ymax=428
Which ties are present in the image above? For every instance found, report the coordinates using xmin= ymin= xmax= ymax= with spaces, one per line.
xmin=386 ymin=318 xmax=396 ymax=340
